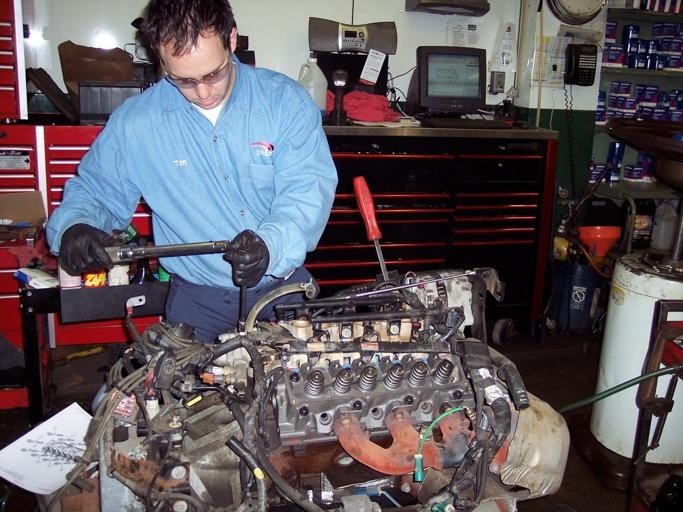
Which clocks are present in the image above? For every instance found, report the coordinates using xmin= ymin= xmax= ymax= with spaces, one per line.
xmin=546 ymin=0 xmax=607 ymax=25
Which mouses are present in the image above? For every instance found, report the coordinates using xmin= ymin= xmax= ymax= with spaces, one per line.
xmin=512 ymin=120 xmax=529 ymax=130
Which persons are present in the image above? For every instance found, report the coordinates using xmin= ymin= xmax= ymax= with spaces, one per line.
xmin=47 ymin=1 xmax=339 ymax=342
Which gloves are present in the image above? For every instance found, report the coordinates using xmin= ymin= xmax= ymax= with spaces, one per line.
xmin=60 ymin=222 xmax=121 ymax=276
xmin=222 ymin=229 xmax=270 ymax=289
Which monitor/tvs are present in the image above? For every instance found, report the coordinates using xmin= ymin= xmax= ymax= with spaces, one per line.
xmin=416 ymin=46 xmax=486 ymax=119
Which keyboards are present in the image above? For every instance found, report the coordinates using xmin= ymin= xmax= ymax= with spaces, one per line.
xmin=419 ymin=118 xmax=514 ymax=130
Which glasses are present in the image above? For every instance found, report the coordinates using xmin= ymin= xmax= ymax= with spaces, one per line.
xmin=163 ymin=60 xmax=231 ymax=87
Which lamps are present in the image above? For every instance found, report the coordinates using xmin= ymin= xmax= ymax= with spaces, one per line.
xmin=325 ymin=70 xmax=348 ymax=126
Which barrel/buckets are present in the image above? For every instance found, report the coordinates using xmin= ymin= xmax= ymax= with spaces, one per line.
xmin=553 ymin=260 xmax=599 ymax=330
xmin=299 ymin=58 xmax=328 ymax=118
xmin=621 ymin=199 xmax=656 ymax=249
xmin=652 ymin=200 xmax=679 ymax=250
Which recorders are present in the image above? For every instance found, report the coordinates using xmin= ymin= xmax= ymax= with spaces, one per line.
xmin=309 ymin=16 xmax=397 ymax=55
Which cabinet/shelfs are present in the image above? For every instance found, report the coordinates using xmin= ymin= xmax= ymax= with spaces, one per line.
xmin=625 ymin=300 xmax=683 ymax=512
xmin=303 ymin=127 xmax=562 ymax=339
xmin=589 ymin=8 xmax=683 ymax=261
xmin=18 ymin=281 xmax=170 ymax=430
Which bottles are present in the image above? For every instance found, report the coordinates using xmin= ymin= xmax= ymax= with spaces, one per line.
xmin=129 ymin=240 xmax=159 ymax=284
